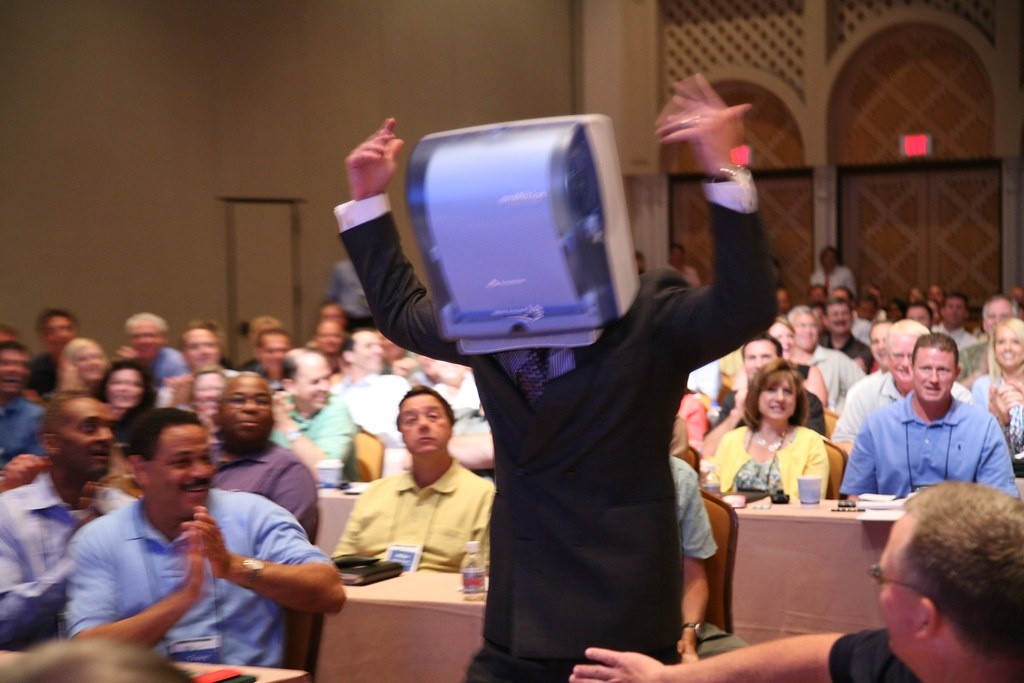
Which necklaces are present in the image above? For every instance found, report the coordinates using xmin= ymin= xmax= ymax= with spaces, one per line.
xmin=755 ymin=429 xmax=786 ymax=452
xmin=906 ymin=422 xmax=952 ymax=492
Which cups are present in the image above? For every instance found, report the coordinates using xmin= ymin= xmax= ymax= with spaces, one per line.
xmin=316 ymin=459 xmax=344 ymax=485
xmin=798 ymin=476 xmax=822 ymax=504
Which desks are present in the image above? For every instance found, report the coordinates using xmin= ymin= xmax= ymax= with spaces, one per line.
xmin=314 ymin=571 xmax=490 ymax=683
xmin=315 ymin=480 xmax=370 ymax=555
xmin=724 ymin=495 xmax=896 ymax=645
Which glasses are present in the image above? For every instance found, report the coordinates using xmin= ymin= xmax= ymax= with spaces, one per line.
xmin=869 ymin=563 xmax=921 ymax=595
xmin=223 ymin=390 xmax=271 ymax=410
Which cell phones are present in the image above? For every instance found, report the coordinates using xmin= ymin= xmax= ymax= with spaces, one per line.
xmin=838 ymin=499 xmax=856 ymax=507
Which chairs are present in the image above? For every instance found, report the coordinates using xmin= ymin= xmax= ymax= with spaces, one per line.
xmin=278 ymin=606 xmax=325 ymax=683
xmin=701 ymin=487 xmax=739 ymax=637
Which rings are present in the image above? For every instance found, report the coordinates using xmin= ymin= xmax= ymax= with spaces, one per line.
xmin=694 ymin=115 xmax=701 ymax=125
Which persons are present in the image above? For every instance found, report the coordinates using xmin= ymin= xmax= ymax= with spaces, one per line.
xmin=568 ymin=482 xmax=1024 ymax=683
xmin=636 ymin=242 xmax=1024 ymax=663
xmin=0 ymin=262 xmax=497 ymax=683
xmin=333 ymin=72 xmax=779 ymax=683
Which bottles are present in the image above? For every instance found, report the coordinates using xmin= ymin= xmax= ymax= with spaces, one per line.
xmin=460 ymin=541 xmax=484 ymax=592
xmin=700 ymin=464 xmax=722 ymax=498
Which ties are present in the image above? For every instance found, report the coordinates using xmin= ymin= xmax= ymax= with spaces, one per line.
xmin=515 ymin=347 xmax=549 ymax=409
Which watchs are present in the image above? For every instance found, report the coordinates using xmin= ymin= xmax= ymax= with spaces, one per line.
xmin=242 ymin=558 xmax=264 ymax=589
xmin=681 ymin=623 xmax=703 ymax=637
